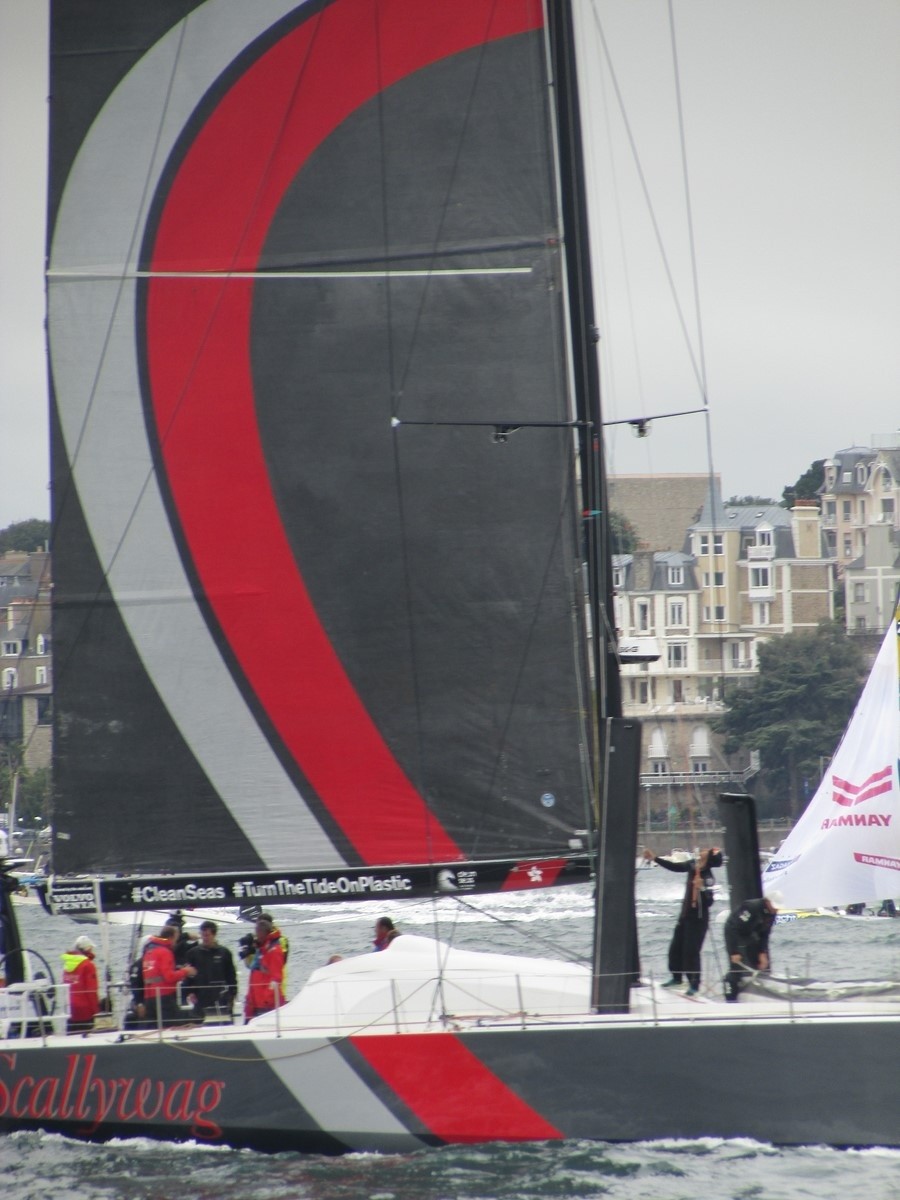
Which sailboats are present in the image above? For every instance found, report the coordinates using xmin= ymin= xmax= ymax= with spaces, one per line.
xmin=634 ymin=750 xmax=776 ymax=869
xmin=761 ymin=614 xmax=900 ymax=925
xmin=0 ymin=0 xmax=900 ymax=1155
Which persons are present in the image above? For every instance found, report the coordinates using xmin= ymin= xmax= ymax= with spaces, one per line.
xmin=371 ymin=917 xmax=402 ymax=953
xmin=642 ymin=846 xmax=723 ymax=997
xmin=721 ymin=891 xmax=785 ymax=1005
xmin=62 ymin=912 xmax=289 ymax=1033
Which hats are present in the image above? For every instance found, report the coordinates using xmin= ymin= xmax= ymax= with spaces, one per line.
xmin=75 ymin=936 xmax=98 ymax=948
xmin=767 ymin=890 xmax=784 ymax=908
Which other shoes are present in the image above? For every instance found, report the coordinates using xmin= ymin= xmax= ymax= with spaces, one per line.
xmin=661 ymin=978 xmax=685 ymax=987
xmin=686 ymin=986 xmax=699 ymax=996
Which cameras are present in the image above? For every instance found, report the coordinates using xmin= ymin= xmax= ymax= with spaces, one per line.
xmin=238 ymin=933 xmax=257 ymax=959
